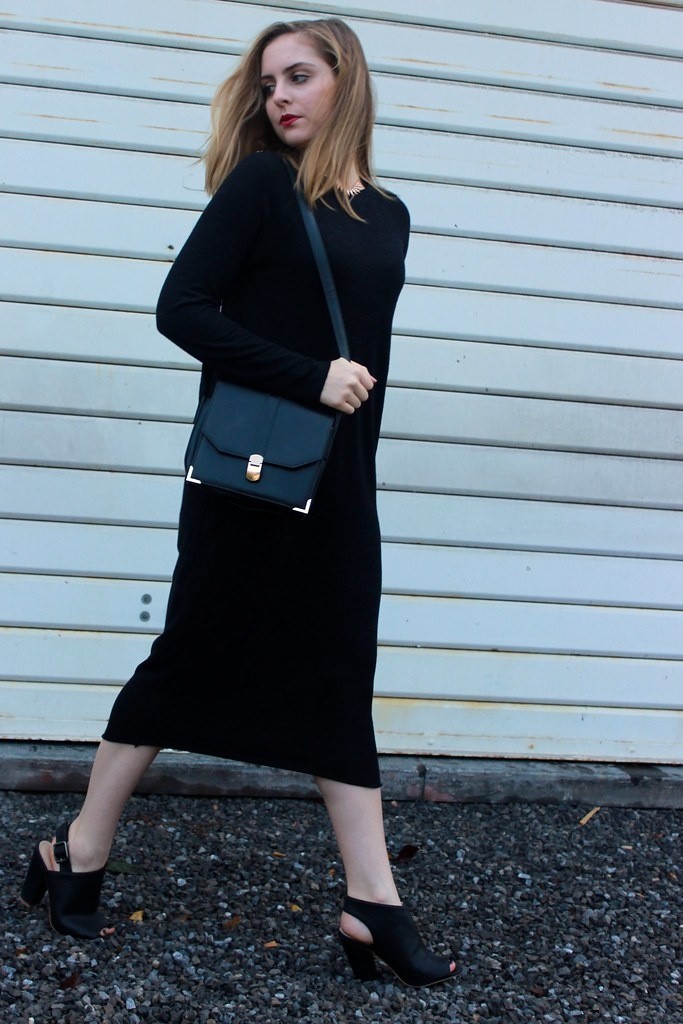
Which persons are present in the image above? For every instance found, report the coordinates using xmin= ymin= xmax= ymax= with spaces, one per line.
xmin=19 ymin=18 xmax=462 ymax=987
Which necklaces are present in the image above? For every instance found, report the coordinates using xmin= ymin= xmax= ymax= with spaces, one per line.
xmin=297 ymin=151 xmax=363 ymax=196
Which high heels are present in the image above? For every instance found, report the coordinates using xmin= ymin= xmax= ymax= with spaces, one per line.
xmin=338 ymin=893 xmax=462 ymax=987
xmin=20 ymin=822 xmax=118 ymax=940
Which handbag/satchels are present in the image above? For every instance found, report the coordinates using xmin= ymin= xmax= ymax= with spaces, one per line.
xmin=184 ymin=381 xmax=337 ymax=515
